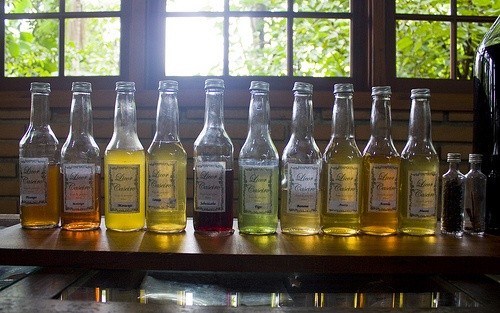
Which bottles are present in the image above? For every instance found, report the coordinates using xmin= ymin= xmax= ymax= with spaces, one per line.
xmin=280 ymin=81 xmax=323 ymax=235
xmin=193 ymin=79 xmax=234 ymax=240
xmin=323 ymin=83 xmax=363 ymax=238
xmin=18 ymin=82 xmax=61 ymax=230
xmin=149 ymin=81 xmax=188 ymax=234
xmin=439 ymin=152 xmax=466 ymax=239
xmin=237 ymin=80 xmax=280 ymax=235
xmin=104 ymin=81 xmax=147 ymax=232
xmin=361 ymin=85 xmax=402 ymax=237
xmin=60 ymin=81 xmax=103 ymax=232
xmin=462 ymin=154 xmax=487 ymax=238
xmin=402 ymin=88 xmax=440 ymax=238
